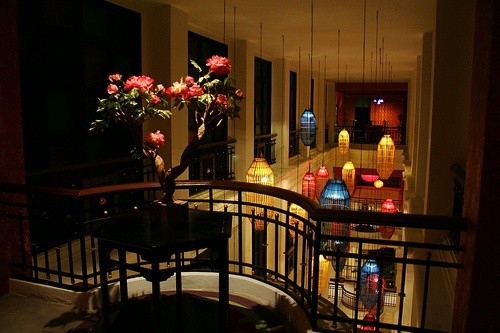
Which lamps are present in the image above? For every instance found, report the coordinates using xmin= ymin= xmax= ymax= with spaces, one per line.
xmin=223 ymin=0 xmax=397 ymax=333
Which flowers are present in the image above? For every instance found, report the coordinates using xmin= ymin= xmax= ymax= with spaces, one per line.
xmin=89 ymin=55 xmax=246 ymax=204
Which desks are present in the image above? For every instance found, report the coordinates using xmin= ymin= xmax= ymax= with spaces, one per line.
xmin=85 ymin=209 xmax=232 ymax=318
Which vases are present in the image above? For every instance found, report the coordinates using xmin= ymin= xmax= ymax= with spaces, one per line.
xmin=151 ymin=201 xmax=188 ymax=226
